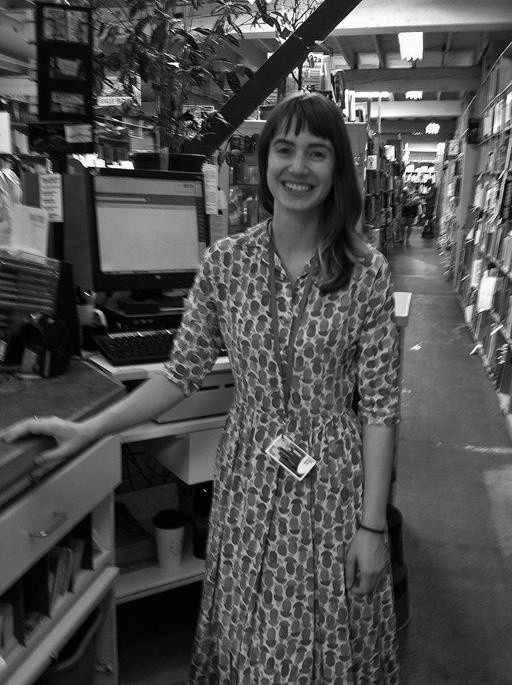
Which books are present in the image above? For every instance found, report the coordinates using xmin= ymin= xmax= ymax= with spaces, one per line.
xmin=360 ymin=86 xmax=512 ymax=426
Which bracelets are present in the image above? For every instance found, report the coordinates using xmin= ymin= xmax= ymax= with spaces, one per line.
xmin=356 ymin=524 xmax=388 ymax=536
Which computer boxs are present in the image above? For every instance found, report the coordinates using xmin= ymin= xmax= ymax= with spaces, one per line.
xmin=93 ymin=300 xmax=184 ymax=330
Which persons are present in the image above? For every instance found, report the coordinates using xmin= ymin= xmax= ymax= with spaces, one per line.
xmin=0 ymin=86 xmax=413 ymax=684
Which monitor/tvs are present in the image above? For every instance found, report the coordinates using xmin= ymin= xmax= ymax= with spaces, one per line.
xmin=84 ymin=166 xmax=211 ymax=313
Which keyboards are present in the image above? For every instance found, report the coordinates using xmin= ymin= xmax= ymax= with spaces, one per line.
xmin=92 ymin=327 xmax=231 ymax=368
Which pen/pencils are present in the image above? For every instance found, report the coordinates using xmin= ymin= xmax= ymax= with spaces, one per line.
xmin=75 ymin=289 xmax=80 ymax=304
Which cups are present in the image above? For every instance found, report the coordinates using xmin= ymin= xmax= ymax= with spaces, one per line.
xmin=150 ymin=508 xmax=188 ymax=573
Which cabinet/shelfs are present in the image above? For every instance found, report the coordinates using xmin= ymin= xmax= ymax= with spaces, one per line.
xmin=0 ymin=355 xmax=233 ymax=685
xmin=432 ymin=42 xmax=511 ymax=427
